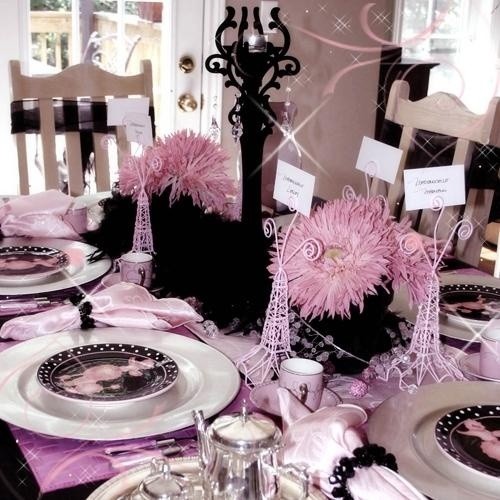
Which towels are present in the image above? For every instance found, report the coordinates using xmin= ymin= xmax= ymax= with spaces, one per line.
xmin=1 ymin=280 xmax=206 ymax=343
xmin=4 ymin=204 xmax=86 ymax=242
xmin=275 ymin=385 xmax=424 ymax=500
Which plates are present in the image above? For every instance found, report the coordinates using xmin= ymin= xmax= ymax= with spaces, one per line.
xmin=104 ymin=274 xmax=161 ymax=293
xmin=366 ymin=379 xmax=500 ymax=499
xmin=458 ymin=352 xmax=500 ymax=382
xmin=1 ymin=238 xmax=112 ymax=297
xmin=249 ymin=380 xmax=344 ymax=419
xmin=0 ymin=326 xmax=239 ymax=444
xmin=434 ymin=282 xmax=500 ymax=323
xmin=387 ymin=274 xmax=500 ymax=341
xmin=432 ymin=403 xmax=500 ymax=478
xmin=84 ymin=455 xmax=326 ymax=500
xmin=0 ymin=245 xmax=72 ymax=281
xmin=34 ymin=342 xmax=178 ymax=407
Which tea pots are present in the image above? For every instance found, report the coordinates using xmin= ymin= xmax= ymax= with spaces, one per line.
xmin=188 ymin=395 xmax=309 ymax=499
xmin=131 ymin=458 xmax=201 ymax=500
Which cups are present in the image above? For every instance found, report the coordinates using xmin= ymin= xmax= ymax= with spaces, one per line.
xmin=279 ymin=356 xmax=326 ymax=414
xmin=480 ymin=329 xmax=500 ymax=377
xmin=119 ymin=254 xmax=154 ymax=288
xmin=58 ymin=202 xmax=88 ymax=235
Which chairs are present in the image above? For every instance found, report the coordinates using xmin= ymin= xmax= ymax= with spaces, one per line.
xmin=362 ymin=79 xmax=499 ymax=269
xmin=7 ymin=29 xmax=159 ymax=195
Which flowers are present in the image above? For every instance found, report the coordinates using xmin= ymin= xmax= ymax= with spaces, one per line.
xmin=113 ymin=129 xmax=244 ymax=221
xmin=264 ymin=195 xmax=415 ymax=326
